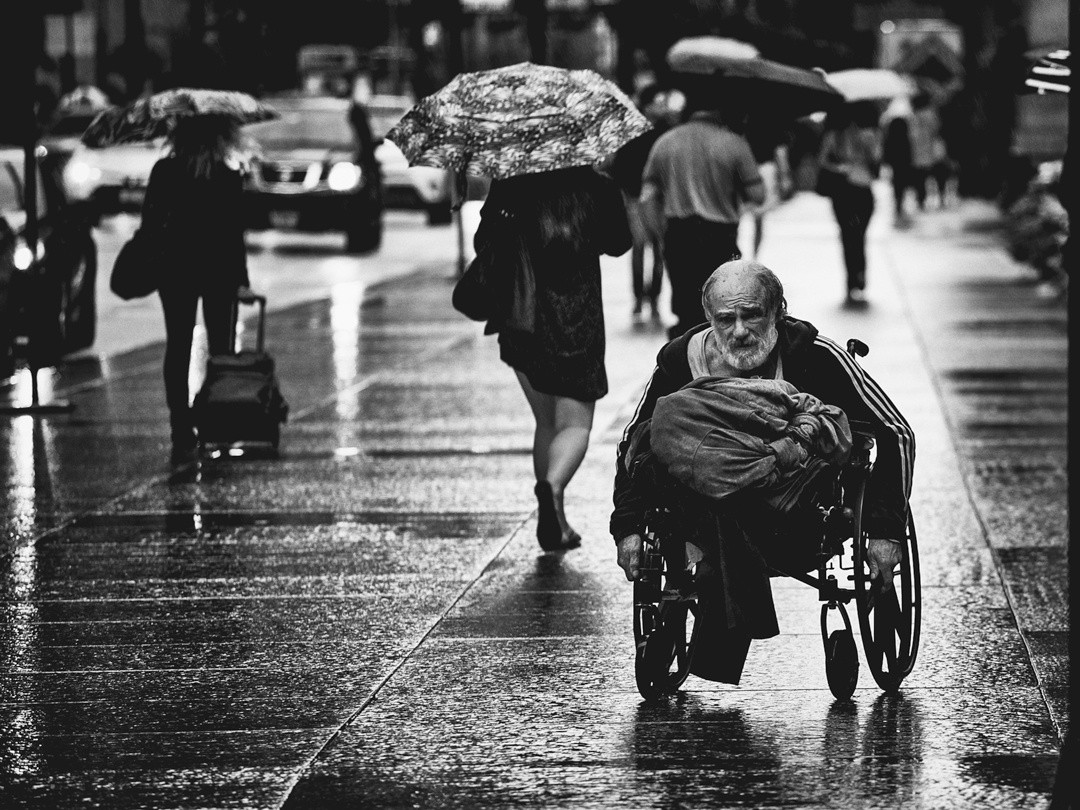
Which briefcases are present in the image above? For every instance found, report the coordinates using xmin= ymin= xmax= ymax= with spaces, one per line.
xmin=194 ymin=297 xmax=287 ymax=459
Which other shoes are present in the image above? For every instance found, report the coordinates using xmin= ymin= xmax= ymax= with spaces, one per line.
xmin=535 ymin=480 xmax=583 ymax=552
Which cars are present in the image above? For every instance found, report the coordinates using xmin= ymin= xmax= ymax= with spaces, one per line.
xmin=30 ymin=104 xmax=182 ymax=227
xmin=0 ymin=145 xmax=97 ymax=376
xmin=238 ymin=93 xmax=387 ymax=255
xmin=366 ymin=94 xmax=460 ymax=225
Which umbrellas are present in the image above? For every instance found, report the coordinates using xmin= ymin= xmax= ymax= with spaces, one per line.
xmin=83 ymin=38 xmax=932 ymax=173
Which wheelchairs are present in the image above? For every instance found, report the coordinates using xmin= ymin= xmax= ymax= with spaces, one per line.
xmin=631 ymin=339 xmax=924 ymax=704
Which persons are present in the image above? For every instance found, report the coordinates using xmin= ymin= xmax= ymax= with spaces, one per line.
xmin=609 ymin=258 xmax=915 ymax=684
xmin=60 ymin=83 xmax=1067 ymax=554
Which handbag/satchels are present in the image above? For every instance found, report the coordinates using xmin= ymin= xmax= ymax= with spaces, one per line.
xmin=885 ymin=118 xmax=911 ymax=166
xmin=813 ymin=154 xmax=848 ymax=196
xmin=110 ymin=228 xmax=157 ymax=299
xmin=483 ymin=244 xmax=536 ymax=335
xmin=650 ymin=377 xmax=853 ymax=500
